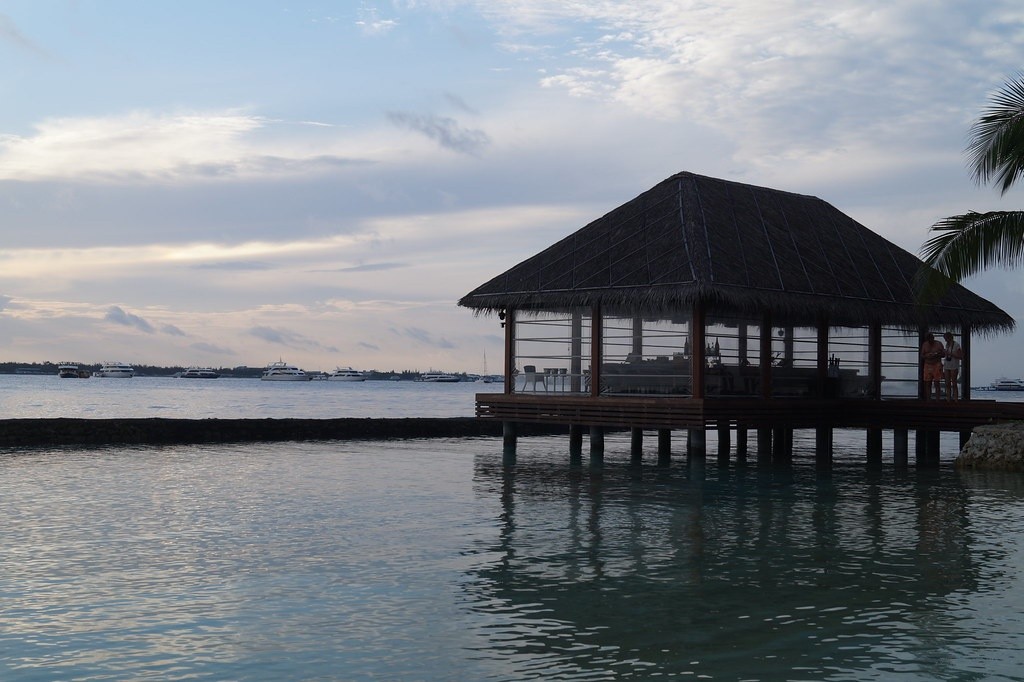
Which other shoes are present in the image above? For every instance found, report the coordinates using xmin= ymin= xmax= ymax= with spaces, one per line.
xmin=947 ymin=400 xmax=952 ymax=405
xmin=926 ymin=400 xmax=931 ymax=404
xmin=954 ymin=399 xmax=958 ymax=405
xmin=935 ymin=400 xmax=942 ymax=405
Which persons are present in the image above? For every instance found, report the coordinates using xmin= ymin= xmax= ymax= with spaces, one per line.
xmin=921 ymin=333 xmax=944 ymax=403
xmin=942 ymin=332 xmax=963 ymax=405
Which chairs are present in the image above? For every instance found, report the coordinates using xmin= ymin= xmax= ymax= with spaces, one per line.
xmin=522 ymin=366 xmax=549 ymax=394
